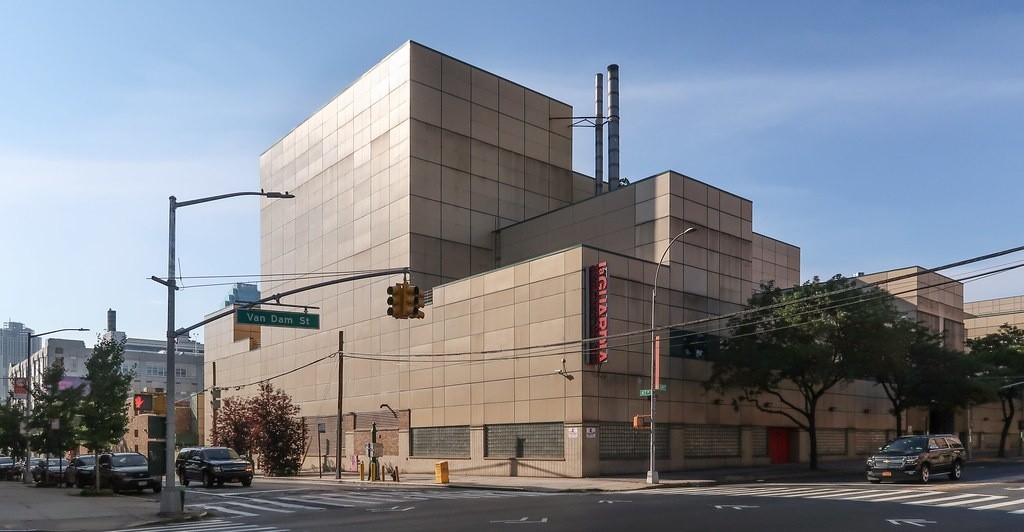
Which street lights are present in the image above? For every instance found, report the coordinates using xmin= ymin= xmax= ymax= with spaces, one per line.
xmin=161 ymin=188 xmax=295 ymax=511
xmin=647 ymin=228 xmax=698 ymax=484
xmin=23 ymin=328 xmax=90 ymax=483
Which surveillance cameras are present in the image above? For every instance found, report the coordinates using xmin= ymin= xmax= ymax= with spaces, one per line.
xmin=556 ymin=370 xmax=562 ymax=373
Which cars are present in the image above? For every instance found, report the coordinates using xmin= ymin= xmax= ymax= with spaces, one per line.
xmin=0 ymin=457 xmax=16 ymax=476
xmin=866 ymin=434 xmax=967 ymax=483
xmin=21 ymin=458 xmax=43 ymax=480
xmin=33 ymin=459 xmax=70 ymax=483
xmin=64 ymin=455 xmax=101 ymax=488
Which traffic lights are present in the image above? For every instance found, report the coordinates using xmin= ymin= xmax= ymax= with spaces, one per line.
xmin=133 ymin=394 xmax=153 ymax=410
xmin=409 ymin=290 xmax=425 ymax=319
xmin=404 ymin=282 xmax=419 ymax=319
xmin=387 ymin=283 xmax=403 ymax=319
xmin=633 ymin=417 xmax=644 ymax=429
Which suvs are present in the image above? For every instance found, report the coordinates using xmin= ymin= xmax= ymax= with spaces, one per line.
xmin=94 ymin=451 xmax=162 ymax=494
xmin=175 ymin=448 xmax=253 ymax=489
